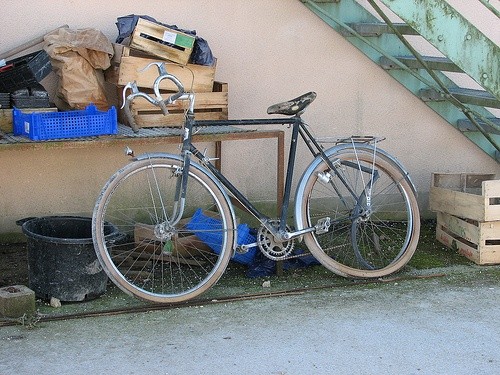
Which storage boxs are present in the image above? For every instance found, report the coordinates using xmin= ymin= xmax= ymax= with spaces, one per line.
xmin=0 ymin=14 xmax=229 ymax=141
xmin=428 ymin=171 xmax=500 ymax=265
xmin=134 ymin=207 xmax=258 ymax=266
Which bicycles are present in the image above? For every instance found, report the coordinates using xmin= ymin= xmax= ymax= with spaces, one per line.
xmin=91 ymin=61 xmax=420 ymax=304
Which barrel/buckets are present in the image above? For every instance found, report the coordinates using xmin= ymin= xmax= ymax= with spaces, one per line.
xmin=16 ymin=214 xmax=127 ymax=304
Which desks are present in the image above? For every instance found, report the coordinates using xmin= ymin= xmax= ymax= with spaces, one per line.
xmin=0 ymin=119 xmax=285 ymax=276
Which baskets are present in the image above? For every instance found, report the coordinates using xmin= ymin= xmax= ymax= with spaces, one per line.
xmin=12 ymin=103 xmax=118 ymax=141
xmin=188 ymin=207 xmax=258 ymax=265
